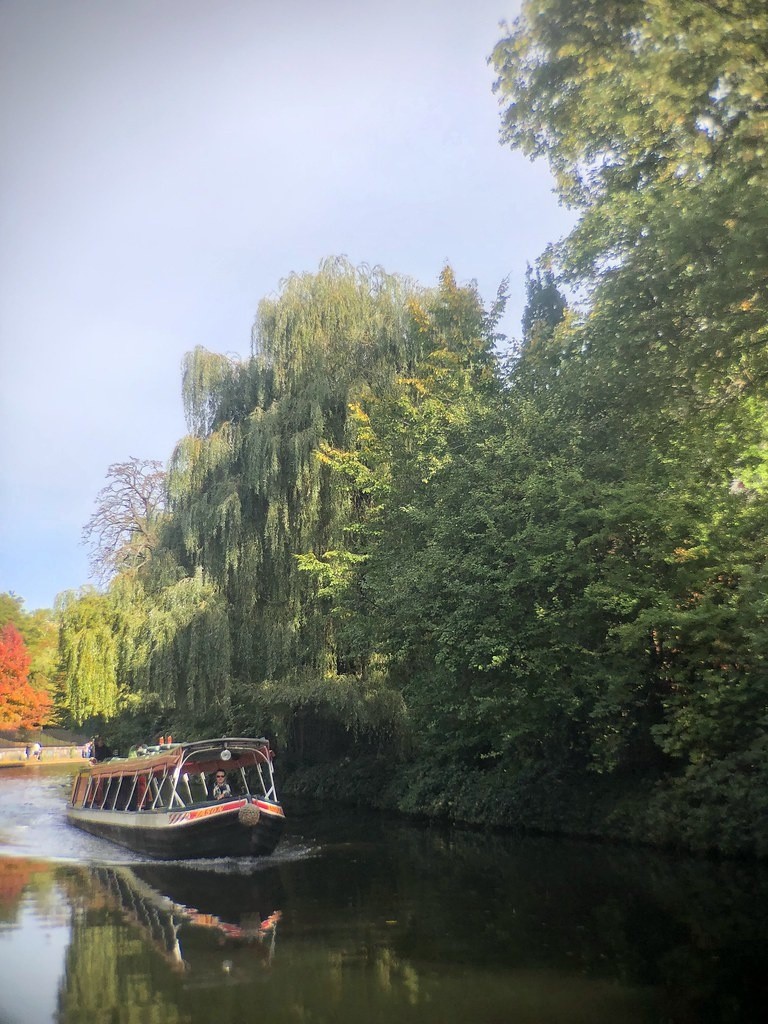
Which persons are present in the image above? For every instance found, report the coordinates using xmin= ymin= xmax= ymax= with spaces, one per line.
xmin=25 ymin=741 xmax=42 ymax=761
xmin=207 ymin=769 xmax=237 ymax=802
xmin=82 ymin=738 xmax=148 ymax=764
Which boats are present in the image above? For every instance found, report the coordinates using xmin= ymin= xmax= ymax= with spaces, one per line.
xmin=60 ymin=855 xmax=284 ymax=1002
xmin=0 ymin=745 xmax=94 ymax=768
xmin=64 ymin=736 xmax=287 ymax=859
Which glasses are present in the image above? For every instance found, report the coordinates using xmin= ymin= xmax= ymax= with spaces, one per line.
xmin=216 ymin=775 xmax=226 ymax=778
